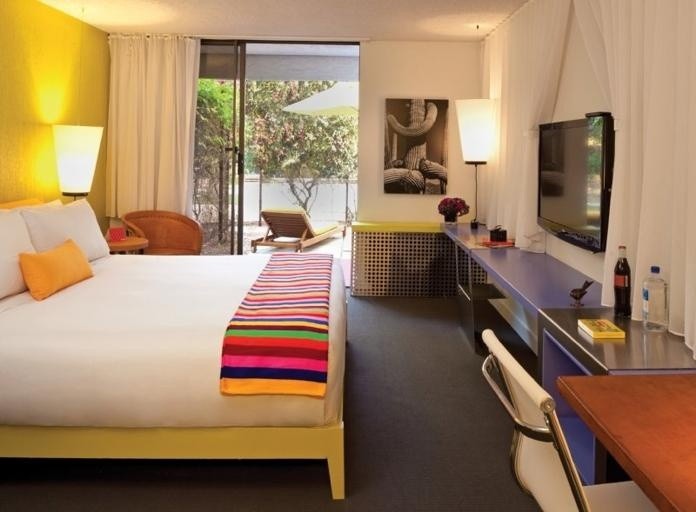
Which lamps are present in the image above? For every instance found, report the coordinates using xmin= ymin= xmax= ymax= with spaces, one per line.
xmin=53 ymin=124 xmax=104 ymax=201
xmin=454 ymin=98 xmax=499 ymax=218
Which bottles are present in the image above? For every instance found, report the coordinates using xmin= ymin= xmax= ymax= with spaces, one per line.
xmin=613 ymin=246 xmax=631 ymax=319
xmin=643 ymin=266 xmax=668 ymax=332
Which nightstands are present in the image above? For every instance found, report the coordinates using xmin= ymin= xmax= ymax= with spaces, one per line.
xmin=105 ymin=236 xmax=148 ymax=255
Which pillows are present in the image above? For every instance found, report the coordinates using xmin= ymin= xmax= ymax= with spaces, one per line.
xmin=0 ymin=210 xmax=36 ymax=299
xmin=19 ymin=239 xmax=93 ymax=299
xmin=18 ymin=199 xmax=109 ymax=265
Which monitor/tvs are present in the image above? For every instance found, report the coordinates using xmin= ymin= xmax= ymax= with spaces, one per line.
xmin=537 ymin=111 xmax=616 ymax=253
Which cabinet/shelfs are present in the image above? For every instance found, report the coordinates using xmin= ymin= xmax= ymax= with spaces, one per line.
xmin=442 ymin=224 xmax=696 ymax=485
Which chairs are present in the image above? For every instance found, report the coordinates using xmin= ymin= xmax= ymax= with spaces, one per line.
xmin=479 ymin=328 xmax=662 ymax=512
xmin=122 ymin=210 xmax=203 ymax=255
xmin=251 ymin=208 xmax=346 ymax=253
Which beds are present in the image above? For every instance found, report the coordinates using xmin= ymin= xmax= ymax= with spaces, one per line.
xmin=0 ymin=198 xmax=346 ymax=500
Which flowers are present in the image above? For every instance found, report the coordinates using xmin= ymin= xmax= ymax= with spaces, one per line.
xmin=437 ymin=198 xmax=470 ymax=217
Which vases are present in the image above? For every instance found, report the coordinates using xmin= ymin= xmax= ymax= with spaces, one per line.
xmin=445 ymin=215 xmax=457 ymax=223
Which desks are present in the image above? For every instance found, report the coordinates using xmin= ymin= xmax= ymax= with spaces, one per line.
xmin=559 ymin=375 xmax=696 ymax=512
